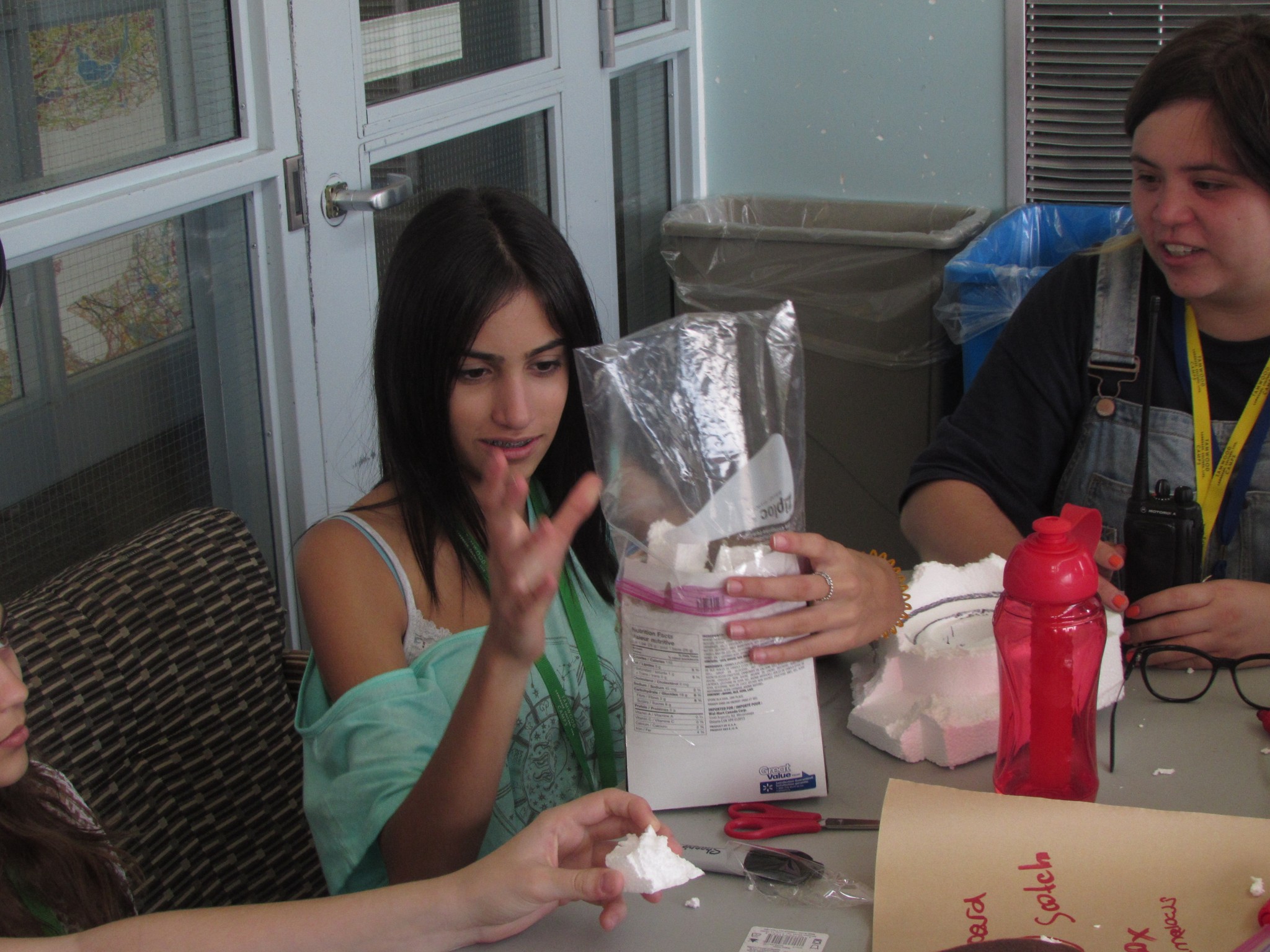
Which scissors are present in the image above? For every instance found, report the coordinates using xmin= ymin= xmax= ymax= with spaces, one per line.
xmin=724 ymin=802 xmax=880 ymax=839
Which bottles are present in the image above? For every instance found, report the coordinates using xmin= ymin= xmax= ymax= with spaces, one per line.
xmin=991 ymin=503 xmax=1108 ymax=804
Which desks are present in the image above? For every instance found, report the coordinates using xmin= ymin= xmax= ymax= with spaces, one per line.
xmin=464 ymin=565 xmax=1270 ymax=952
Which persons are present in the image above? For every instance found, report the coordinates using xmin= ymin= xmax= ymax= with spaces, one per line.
xmin=0 ymin=605 xmax=681 ymax=952
xmin=292 ymin=185 xmax=911 ymax=884
xmin=895 ymin=13 xmax=1270 ymax=673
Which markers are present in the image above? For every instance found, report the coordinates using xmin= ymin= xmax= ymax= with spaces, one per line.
xmin=681 ymin=845 xmax=825 ymax=884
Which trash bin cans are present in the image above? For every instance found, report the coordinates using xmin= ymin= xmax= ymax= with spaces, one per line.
xmin=947 ymin=203 xmax=1138 ymax=389
xmin=664 ymin=194 xmax=991 ymax=569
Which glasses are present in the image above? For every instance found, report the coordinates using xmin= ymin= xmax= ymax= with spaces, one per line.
xmin=1110 ymin=644 xmax=1270 ymax=775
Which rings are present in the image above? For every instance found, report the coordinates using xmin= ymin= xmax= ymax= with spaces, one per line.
xmin=811 ymin=571 xmax=834 ymax=602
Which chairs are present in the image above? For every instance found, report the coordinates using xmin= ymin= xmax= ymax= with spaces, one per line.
xmin=0 ymin=501 xmax=311 ymax=915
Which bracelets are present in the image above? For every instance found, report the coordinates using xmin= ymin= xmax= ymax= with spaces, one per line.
xmin=858 ymin=549 xmax=910 ymax=642
